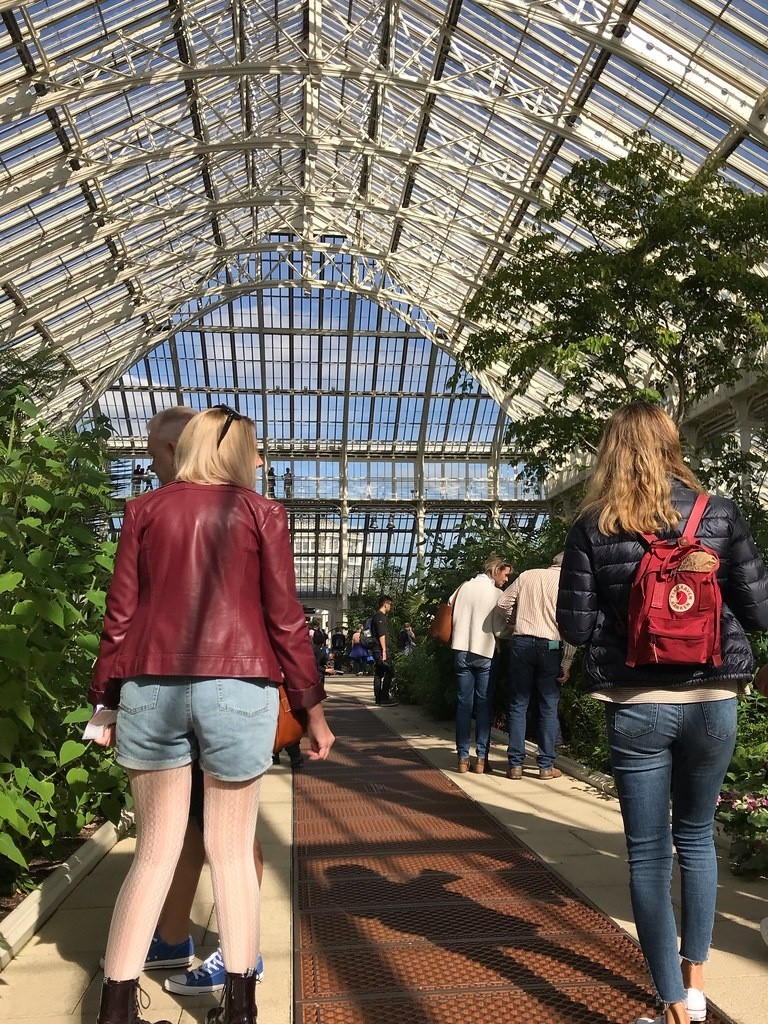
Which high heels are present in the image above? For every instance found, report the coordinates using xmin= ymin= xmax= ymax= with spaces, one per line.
xmin=458 ymin=759 xmax=473 ymax=773
xmin=475 ymin=758 xmax=493 ymax=773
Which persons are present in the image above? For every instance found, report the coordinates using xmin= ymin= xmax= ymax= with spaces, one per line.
xmin=496 ymin=551 xmax=578 ymax=779
xmin=87 ymin=405 xmax=336 ymax=1024
xmin=98 ymin=407 xmax=263 ymax=996
xmin=397 ymin=622 xmax=415 ymax=657
xmin=331 ymin=628 xmax=346 ymax=675
xmin=349 ymin=624 xmax=367 ymax=676
xmin=556 ymin=401 xmax=768 ymax=1024
xmin=370 ymin=595 xmax=399 ymax=707
xmin=447 ymin=554 xmax=515 ymax=775
xmin=310 ymin=624 xmax=330 ymax=685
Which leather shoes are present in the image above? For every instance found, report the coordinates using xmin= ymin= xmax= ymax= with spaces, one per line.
xmin=508 ymin=767 xmax=523 ymax=779
xmin=539 ymin=767 xmax=562 ymax=780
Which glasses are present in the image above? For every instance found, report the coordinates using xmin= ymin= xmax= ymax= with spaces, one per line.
xmin=386 ymin=602 xmax=393 ymax=607
xmin=213 ymin=403 xmax=242 ymax=450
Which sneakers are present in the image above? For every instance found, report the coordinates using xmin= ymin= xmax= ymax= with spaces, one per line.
xmin=375 ymin=699 xmax=381 ymax=706
xmin=100 ymin=925 xmax=194 ymax=970
xmin=165 ymin=948 xmax=263 ymax=995
xmin=381 ymin=700 xmax=399 ymax=706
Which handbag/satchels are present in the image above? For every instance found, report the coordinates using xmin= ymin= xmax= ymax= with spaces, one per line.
xmin=428 ymin=604 xmax=453 ymax=646
xmin=273 ymin=684 xmax=302 ymax=752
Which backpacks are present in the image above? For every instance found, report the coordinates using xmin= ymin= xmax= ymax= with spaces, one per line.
xmin=335 ymin=639 xmax=344 ymax=650
xmin=360 ymin=619 xmax=373 ymax=650
xmin=627 ymin=494 xmax=723 ymax=668
xmin=396 ymin=631 xmax=409 ymax=649
xmin=313 ymin=631 xmax=324 ymax=645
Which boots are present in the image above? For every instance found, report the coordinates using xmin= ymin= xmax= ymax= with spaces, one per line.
xmin=207 ymin=967 xmax=258 ymax=1024
xmin=97 ymin=976 xmax=173 ymax=1024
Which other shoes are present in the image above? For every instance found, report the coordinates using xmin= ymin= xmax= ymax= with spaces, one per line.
xmin=683 ymin=988 xmax=707 ymax=1021
xmin=635 ymin=1016 xmax=664 ymax=1024
xmin=355 ymin=672 xmax=369 ymax=677
